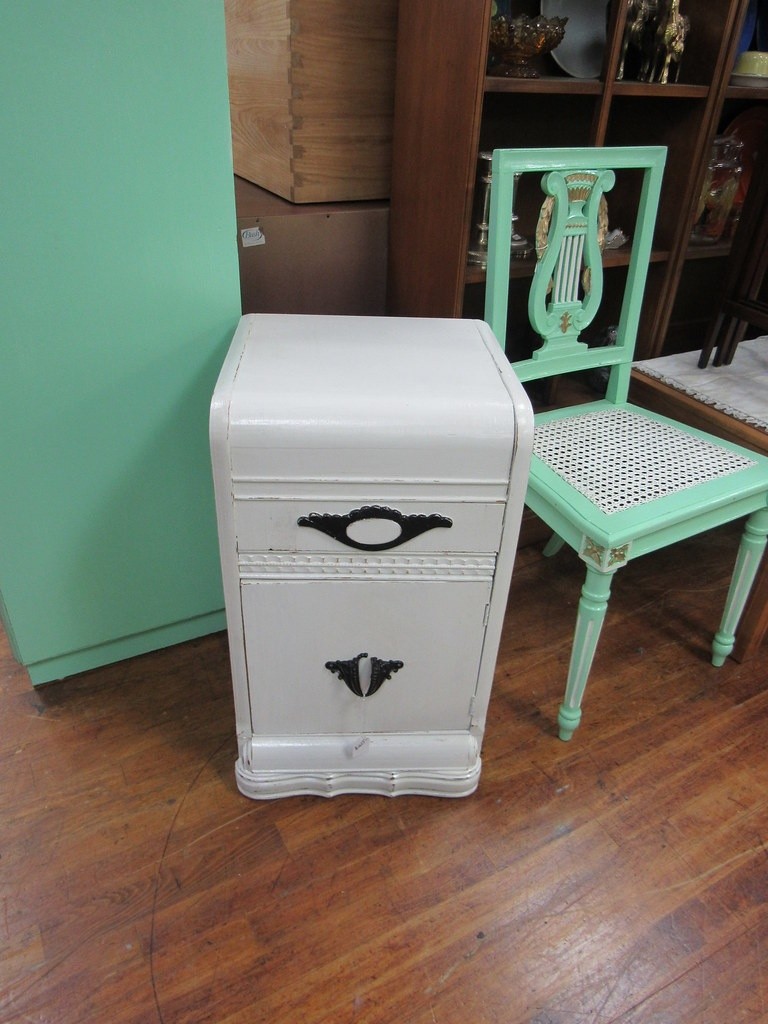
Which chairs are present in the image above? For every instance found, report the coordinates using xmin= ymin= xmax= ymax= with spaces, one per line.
xmin=484 ymin=147 xmax=768 ymax=739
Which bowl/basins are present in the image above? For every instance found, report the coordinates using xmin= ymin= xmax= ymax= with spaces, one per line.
xmin=732 ymin=51 xmax=768 ymax=77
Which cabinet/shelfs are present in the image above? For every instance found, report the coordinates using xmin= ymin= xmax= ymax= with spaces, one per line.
xmin=386 ymin=0 xmax=737 ymax=405
xmin=653 ymin=0 xmax=768 ymax=355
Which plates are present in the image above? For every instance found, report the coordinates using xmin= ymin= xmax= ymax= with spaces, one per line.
xmin=489 ymin=14 xmax=569 ymax=78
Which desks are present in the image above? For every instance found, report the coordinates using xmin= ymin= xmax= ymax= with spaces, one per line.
xmin=630 ymin=333 xmax=768 ymax=457
